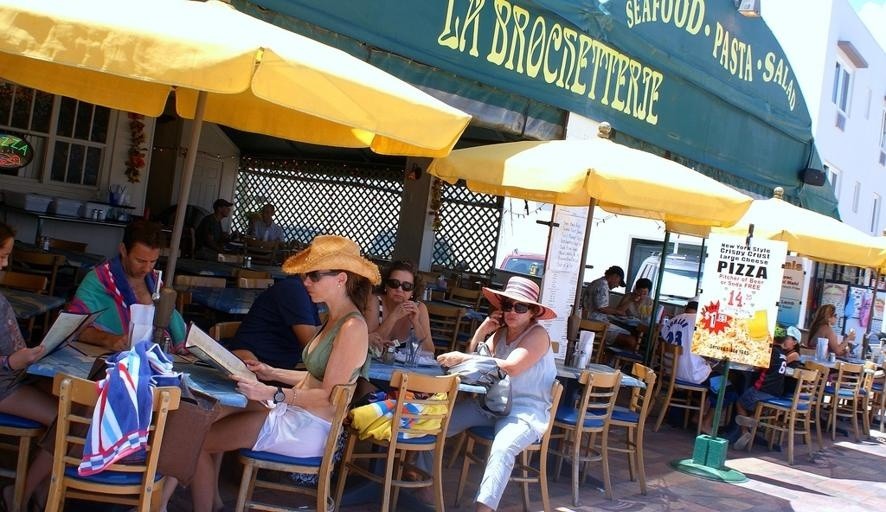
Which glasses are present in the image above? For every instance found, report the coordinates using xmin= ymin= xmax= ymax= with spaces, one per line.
xmin=297 ymin=271 xmax=340 ymax=283
xmin=385 ymin=278 xmax=416 ymax=292
xmin=500 ymin=301 xmax=534 ymax=313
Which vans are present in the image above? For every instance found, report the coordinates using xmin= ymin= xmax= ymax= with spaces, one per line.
xmin=629 ymin=251 xmax=706 ymax=306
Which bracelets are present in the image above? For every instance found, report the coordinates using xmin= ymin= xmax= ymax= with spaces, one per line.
xmin=6 ymin=350 xmax=16 ymax=372
xmin=290 ymin=388 xmax=297 ymax=406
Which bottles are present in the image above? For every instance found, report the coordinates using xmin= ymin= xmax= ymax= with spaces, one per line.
xmin=570 ymin=349 xmax=587 ymax=370
xmin=383 ymin=347 xmax=394 ymax=364
xmin=828 ymin=353 xmax=836 ymax=362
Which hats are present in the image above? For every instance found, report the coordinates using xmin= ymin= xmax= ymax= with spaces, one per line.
xmin=214 ymin=199 xmax=233 ymax=209
xmin=609 ymin=266 xmax=627 ymax=287
xmin=282 ymin=236 xmax=383 ymax=286
xmin=774 ymin=325 xmax=802 ymax=344
xmin=482 ymin=276 xmax=557 ymax=320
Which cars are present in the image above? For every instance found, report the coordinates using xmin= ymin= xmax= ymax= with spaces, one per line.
xmin=370 ymin=229 xmax=455 ymax=271
xmin=500 ymin=254 xmax=547 ymax=277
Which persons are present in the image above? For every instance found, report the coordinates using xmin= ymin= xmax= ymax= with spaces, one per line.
xmin=69 ymin=221 xmax=192 ymax=357
xmin=193 ymin=198 xmax=240 ymax=259
xmin=662 ymin=296 xmax=804 ymax=450
xmin=189 ymin=235 xmax=383 ymax=512
xmin=0 ymin=222 xmax=74 ymax=512
xmin=229 ymin=269 xmax=324 ymax=387
xmin=402 ymin=275 xmax=557 ymax=512
xmin=361 ymin=256 xmax=435 ymax=469
xmin=581 ymin=266 xmax=654 ymax=350
xmin=808 ymin=304 xmax=857 ymax=357
xmin=245 ymin=204 xmax=285 ymax=249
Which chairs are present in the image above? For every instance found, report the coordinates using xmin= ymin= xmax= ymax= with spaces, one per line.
xmin=549 ymin=368 xmax=625 ymax=506
xmin=450 ymin=378 xmax=567 ymax=511
xmin=46 ymin=368 xmax=183 ymax=512
xmin=584 ymin=362 xmax=657 ymax=498
xmin=652 ymin=327 xmax=886 ymax=456
xmin=231 ymin=381 xmax=361 ymax=511
xmin=335 ymin=370 xmax=464 ymax=512
xmin=1 ymin=410 xmax=45 ymax=511
xmin=0 ymin=228 xmax=648 ymax=387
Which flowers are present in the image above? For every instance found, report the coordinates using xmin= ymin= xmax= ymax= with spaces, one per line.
xmin=122 ymin=112 xmax=149 ymax=184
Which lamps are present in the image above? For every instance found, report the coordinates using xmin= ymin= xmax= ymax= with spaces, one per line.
xmin=735 ymin=0 xmax=762 ymax=18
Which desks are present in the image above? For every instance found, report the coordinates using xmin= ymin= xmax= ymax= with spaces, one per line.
xmin=29 ymin=212 xmax=127 ymax=246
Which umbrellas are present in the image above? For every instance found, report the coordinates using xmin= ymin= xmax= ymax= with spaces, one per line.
xmin=2 ymin=0 xmax=475 ymax=354
xmin=795 ymin=230 xmax=886 ymax=360
xmin=665 ymin=185 xmax=886 ymax=271
xmin=426 ymin=120 xmax=756 ymax=355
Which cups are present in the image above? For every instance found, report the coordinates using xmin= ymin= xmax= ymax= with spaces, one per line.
xmin=405 ymin=335 xmax=422 ymax=368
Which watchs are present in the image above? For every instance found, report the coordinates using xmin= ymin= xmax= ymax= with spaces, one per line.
xmin=273 ymin=386 xmax=285 ymax=404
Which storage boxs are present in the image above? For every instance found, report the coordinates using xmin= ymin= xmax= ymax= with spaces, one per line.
xmin=54 ymin=196 xmax=82 ymax=217
xmin=22 ymin=192 xmax=53 ymax=212
xmin=78 ymin=200 xmax=110 ymax=221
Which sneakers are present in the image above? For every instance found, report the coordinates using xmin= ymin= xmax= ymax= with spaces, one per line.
xmin=735 ymin=415 xmax=755 ymax=428
xmin=732 ymin=432 xmax=751 ymax=450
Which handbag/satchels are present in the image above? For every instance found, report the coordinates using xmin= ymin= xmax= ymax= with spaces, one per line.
xmin=475 ymin=375 xmax=513 ymax=419
